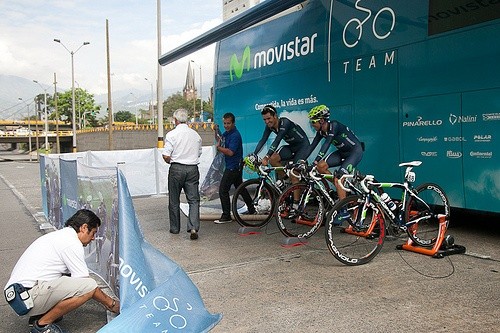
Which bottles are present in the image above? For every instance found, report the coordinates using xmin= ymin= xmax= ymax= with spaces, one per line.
xmin=379 ymin=188 xmax=397 ymax=211
xmin=276 ymin=180 xmax=286 ymax=191
xmin=329 ymin=189 xmax=339 ymax=202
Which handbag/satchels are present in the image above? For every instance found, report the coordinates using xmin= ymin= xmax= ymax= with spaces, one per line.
xmin=4 ymin=282 xmax=35 ymax=316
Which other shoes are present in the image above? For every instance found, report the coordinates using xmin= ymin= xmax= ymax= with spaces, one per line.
xmin=288 ymin=208 xmax=302 ymax=218
xmin=191 ymin=229 xmax=197 ymax=239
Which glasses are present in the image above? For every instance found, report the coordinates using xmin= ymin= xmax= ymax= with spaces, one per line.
xmin=309 ymin=119 xmax=320 ymax=124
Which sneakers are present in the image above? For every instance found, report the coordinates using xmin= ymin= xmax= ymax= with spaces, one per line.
xmin=213 ymin=218 xmax=233 ymax=224
xmin=242 ymin=210 xmax=257 ymax=215
xmin=29 ymin=313 xmax=63 ymax=327
xmin=334 ymin=212 xmax=351 ymax=224
xmin=29 ymin=320 xmax=66 ymax=333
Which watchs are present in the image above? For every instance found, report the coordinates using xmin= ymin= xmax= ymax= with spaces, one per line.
xmin=109 ymin=299 xmax=116 ymax=308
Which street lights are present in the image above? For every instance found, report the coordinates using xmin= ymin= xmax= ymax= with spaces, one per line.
xmin=130 ymin=92 xmax=139 ymax=124
xmin=74 ymin=80 xmax=82 ymax=129
xmin=54 ymin=38 xmax=91 ymax=153
xmin=33 ymin=79 xmax=58 ymax=154
xmin=18 ymin=96 xmax=39 ymax=162
xmin=143 ymin=77 xmax=156 ymax=124
xmin=191 ymin=59 xmax=204 ymax=122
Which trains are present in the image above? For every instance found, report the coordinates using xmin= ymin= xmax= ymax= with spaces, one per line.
xmin=0 ymin=120 xmax=80 ymax=132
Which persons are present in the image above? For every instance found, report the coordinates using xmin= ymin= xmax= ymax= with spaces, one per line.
xmin=298 ymin=105 xmax=364 ymax=224
xmin=162 ymin=108 xmax=201 ymax=241
xmin=3 ymin=209 xmax=121 ymax=333
xmin=250 ymin=105 xmax=309 ymax=218
xmin=214 ymin=113 xmax=256 ymax=224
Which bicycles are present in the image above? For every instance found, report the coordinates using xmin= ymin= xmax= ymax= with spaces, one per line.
xmin=231 ymin=152 xmax=384 ymax=242
xmin=324 ymin=160 xmax=451 ymax=266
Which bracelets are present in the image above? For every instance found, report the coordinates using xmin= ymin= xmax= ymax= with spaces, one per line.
xmin=266 ymin=156 xmax=269 ymax=159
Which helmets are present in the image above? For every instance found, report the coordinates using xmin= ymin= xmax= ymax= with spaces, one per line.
xmin=307 ymin=105 xmax=330 ymax=120
xmin=244 ymin=157 xmax=256 ymax=172
